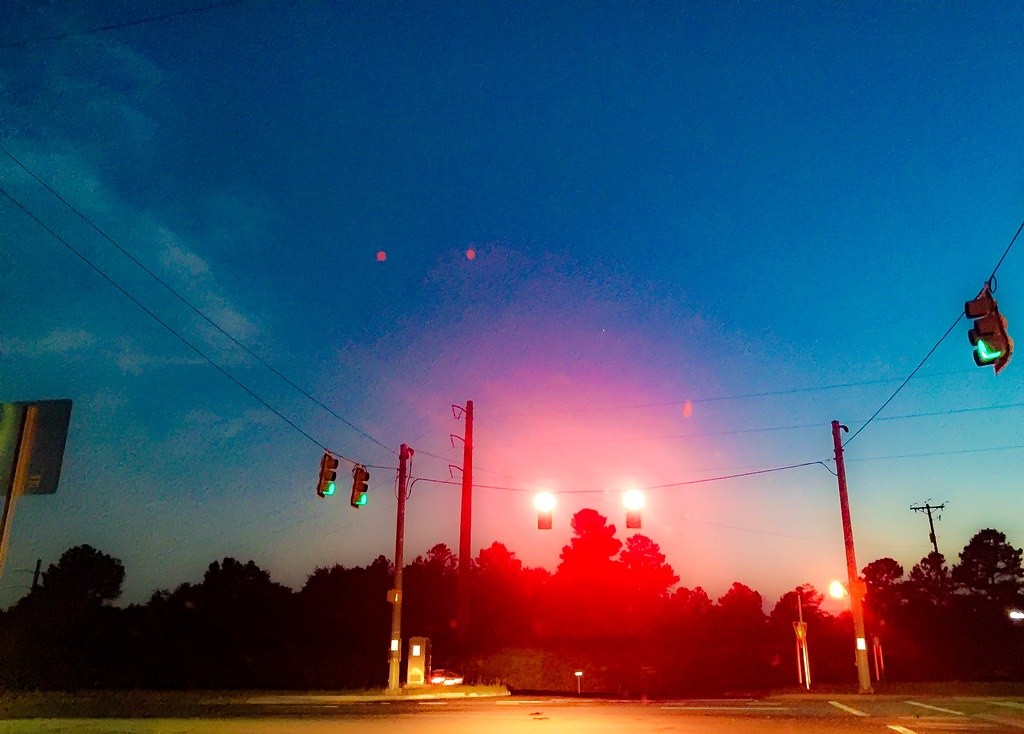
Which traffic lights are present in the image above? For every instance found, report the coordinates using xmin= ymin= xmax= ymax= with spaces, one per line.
xmin=626 ymin=491 xmax=641 ymax=529
xmin=317 ymin=454 xmax=339 ymax=499
xmin=351 ymin=467 xmax=371 ymax=508
xmin=966 ymin=289 xmax=1011 ymax=374
xmin=537 ymin=492 xmax=553 ymax=530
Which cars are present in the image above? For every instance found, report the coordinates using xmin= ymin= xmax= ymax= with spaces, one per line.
xmin=432 ymin=669 xmax=464 ymax=685
